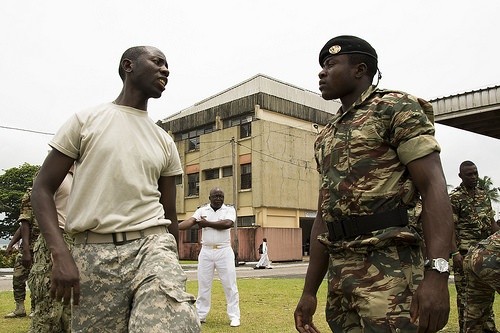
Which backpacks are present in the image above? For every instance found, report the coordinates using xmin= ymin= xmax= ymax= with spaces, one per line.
xmin=259 ymin=243 xmax=263 ymax=254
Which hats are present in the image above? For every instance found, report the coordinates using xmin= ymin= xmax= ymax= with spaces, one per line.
xmin=319 ymin=34 xmax=378 ymax=66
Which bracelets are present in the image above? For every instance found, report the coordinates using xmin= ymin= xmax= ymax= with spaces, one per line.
xmin=451 ymin=251 xmax=460 ymax=256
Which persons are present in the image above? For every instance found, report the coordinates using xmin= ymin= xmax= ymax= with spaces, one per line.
xmin=463 ymin=229 xmax=500 ymax=333
xmin=447 ymin=161 xmax=499 ymax=333
xmin=179 ymin=187 xmax=241 ymax=327
xmin=31 ymin=45 xmax=201 ymax=332
xmin=256 ymin=238 xmax=273 ymax=269
xmin=6 ymin=161 xmax=73 ymax=331
xmin=294 ymin=36 xmax=454 ymax=333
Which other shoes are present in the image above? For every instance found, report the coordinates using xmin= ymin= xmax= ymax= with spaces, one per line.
xmin=230 ymin=319 xmax=241 ymax=327
xmin=200 ymin=318 xmax=207 ymax=323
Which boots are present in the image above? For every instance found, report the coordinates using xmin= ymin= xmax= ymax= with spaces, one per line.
xmin=4 ymin=301 xmax=26 ymax=318
xmin=28 ymin=306 xmax=36 ymax=317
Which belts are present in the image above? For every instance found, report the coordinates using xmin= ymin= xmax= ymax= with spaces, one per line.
xmin=73 ymin=225 xmax=169 ymax=246
xmin=202 ymin=245 xmax=222 ymax=249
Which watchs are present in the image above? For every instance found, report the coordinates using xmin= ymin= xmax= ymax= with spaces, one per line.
xmin=424 ymin=258 xmax=452 ymax=274
